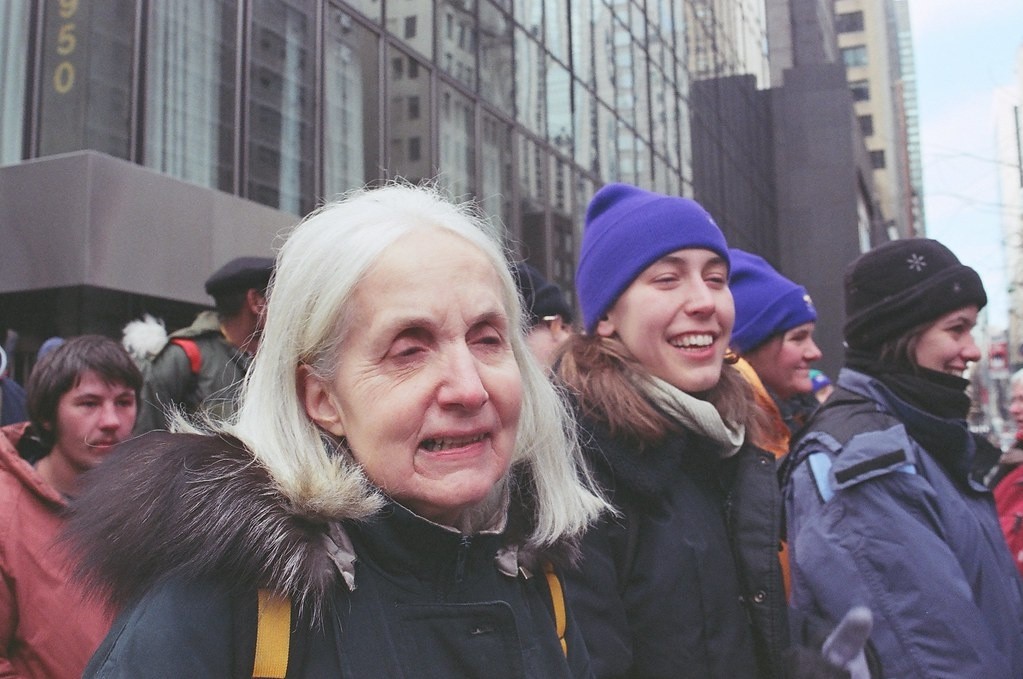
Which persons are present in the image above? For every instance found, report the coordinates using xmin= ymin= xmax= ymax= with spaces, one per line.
xmin=552 ymin=185 xmax=878 ymax=679
xmin=1 ymin=334 xmax=142 ymax=679
xmin=989 ymin=368 xmax=1023 ymax=570
xmin=810 ymin=371 xmax=833 ymax=403
xmin=506 ymin=263 xmax=572 ymax=370
xmin=786 ymin=239 xmax=1023 ymax=678
xmin=729 ymin=248 xmax=835 ymax=609
xmin=83 ymin=184 xmax=596 ymax=679
xmin=0 ymin=337 xmax=65 ymax=426
xmin=137 ymin=256 xmax=276 ymax=433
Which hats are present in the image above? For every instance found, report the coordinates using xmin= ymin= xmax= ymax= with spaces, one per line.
xmin=204 ymin=258 xmax=276 ymax=302
xmin=718 ymin=247 xmax=818 ymax=353
xmin=571 ymin=180 xmax=731 ymax=335
xmin=843 ymin=238 xmax=988 ymax=350
xmin=511 ymin=260 xmax=573 ymax=328
